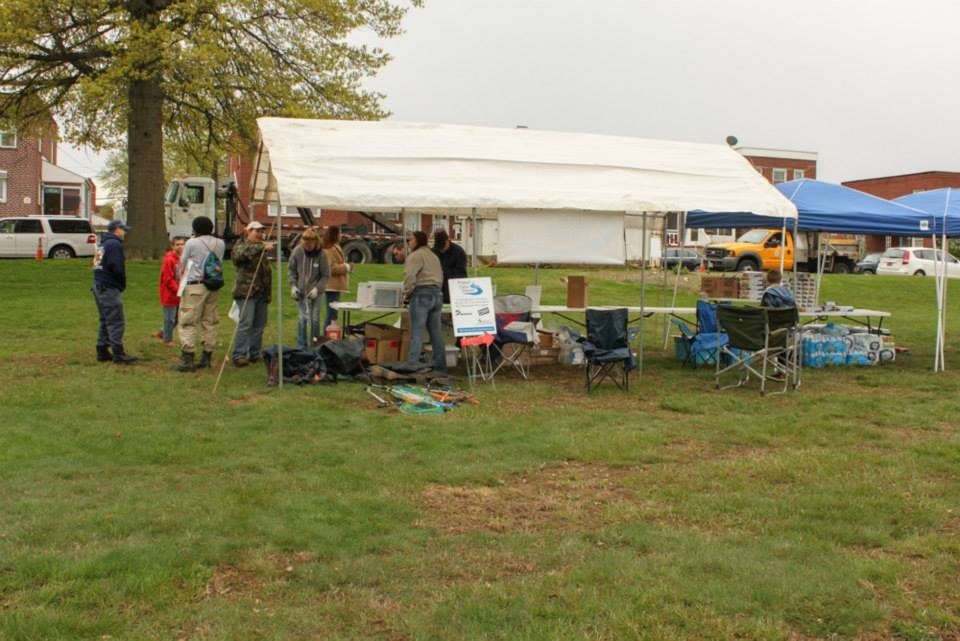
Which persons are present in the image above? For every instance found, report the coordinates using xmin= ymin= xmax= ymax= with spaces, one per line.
xmin=400 ymin=230 xmax=449 ymax=377
xmin=758 ymin=269 xmax=800 ymax=381
xmin=230 ymin=220 xmax=279 ymax=367
xmin=170 ymin=215 xmax=225 ymax=373
xmin=159 ymin=235 xmax=186 ymax=347
xmin=319 ymin=223 xmax=353 ymax=338
xmin=285 ymin=226 xmax=333 ymax=351
xmin=430 ymin=228 xmax=468 ymax=347
xmin=91 ymin=220 xmax=139 ymax=364
xmin=766 ymin=234 xmax=781 ymax=245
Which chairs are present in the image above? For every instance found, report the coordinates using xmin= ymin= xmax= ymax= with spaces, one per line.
xmin=579 ymin=299 xmax=806 ymax=396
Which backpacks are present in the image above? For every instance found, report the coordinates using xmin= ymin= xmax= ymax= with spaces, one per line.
xmin=199 ymin=238 xmax=224 ymax=290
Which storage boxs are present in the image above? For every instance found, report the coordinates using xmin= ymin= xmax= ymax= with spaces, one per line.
xmin=355 ymin=280 xmax=406 ymax=307
xmin=705 ymin=269 xmax=818 ymax=304
xmin=566 ymin=273 xmax=591 ymax=308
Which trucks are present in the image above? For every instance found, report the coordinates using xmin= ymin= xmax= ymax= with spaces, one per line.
xmin=702 ymin=228 xmax=867 ymax=271
xmin=666 ymin=211 xmax=736 ymax=247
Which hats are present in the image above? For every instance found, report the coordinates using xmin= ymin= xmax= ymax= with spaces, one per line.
xmin=433 ymin=229 xmax=448 ymax=248
xmin=108 ymin=220 xmax=132 ymax=232
xmin=247 ymin=221 xmax=265 ymax=231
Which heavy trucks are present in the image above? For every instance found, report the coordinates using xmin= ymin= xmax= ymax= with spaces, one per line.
xmin=161 ymin=129 xmax=666 ymax=271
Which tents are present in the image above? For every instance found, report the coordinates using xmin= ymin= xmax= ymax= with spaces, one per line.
xmin=661 ymin=179 xmax=946 ymax=374
xmin=812 ymin=187 xmax=960 ymax=374
xmin=209 ymin=116 xmax=800 ymax=400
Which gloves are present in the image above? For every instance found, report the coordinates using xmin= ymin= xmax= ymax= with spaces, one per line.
xmin=290 ymin=286 xmax=301 ymax=299
xmin=307 ymin=288 xmax=317 ymax=299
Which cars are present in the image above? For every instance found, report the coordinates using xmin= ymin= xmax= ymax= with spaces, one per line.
xmin=875 ymin=247 xmax=959 ymax=278
xmin=853 ymin=252 xmax=882 ymax=274
xmin=663 ymin=247 xmax=707 ymax=271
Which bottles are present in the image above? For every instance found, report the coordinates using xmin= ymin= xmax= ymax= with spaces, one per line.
xmin=800 ymin=323 xmax=870 ymax=368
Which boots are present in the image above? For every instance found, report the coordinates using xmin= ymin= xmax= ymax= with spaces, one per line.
xmin=194 ymin=351 xmax=212 ymax=369
xmin=178 ymin=351 xmax=194 ymax=372
xmin=96 ymin=345 xmax=112 ymax=361
xmin=111 ymin=343 xmax=137 ymax=362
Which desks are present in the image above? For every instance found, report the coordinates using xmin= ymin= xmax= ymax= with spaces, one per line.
xmin=327 ymin=299 xmax=895 ymax=373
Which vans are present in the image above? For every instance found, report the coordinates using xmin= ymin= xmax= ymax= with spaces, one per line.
xmin=0 ymin=214 xmax=97 ymax=260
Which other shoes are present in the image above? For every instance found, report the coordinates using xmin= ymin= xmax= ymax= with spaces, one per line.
xmin=235 ymin=358 xmax=249 ymax=367
xmin=155 ymin=329 xmax=176 ymax=346
xmin=251 ymin=355 xmax=263 ymax=362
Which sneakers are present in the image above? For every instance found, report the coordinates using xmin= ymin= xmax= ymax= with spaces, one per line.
xmin=766 ymin=373 xmax=787 ymax=382
